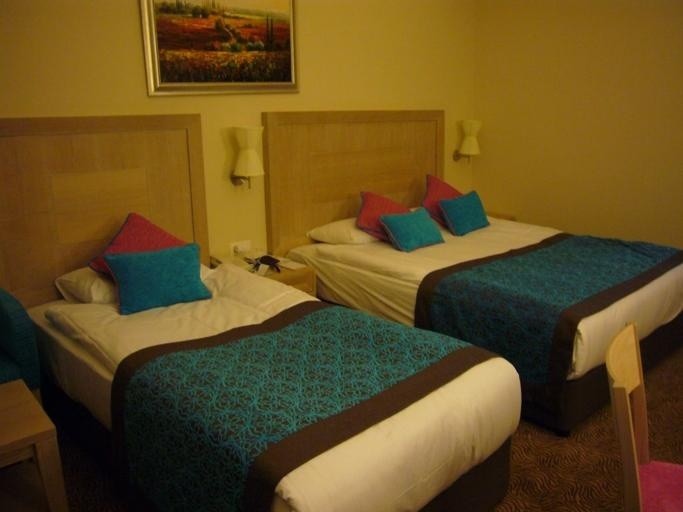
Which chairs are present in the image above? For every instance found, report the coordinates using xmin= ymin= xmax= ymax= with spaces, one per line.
xmin=0 ymin=290 xmax=71 ymax=511
xmin=604 ymin=321 xmax=683 ymax=512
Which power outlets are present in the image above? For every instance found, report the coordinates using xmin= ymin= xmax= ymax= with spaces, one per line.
xmin=229 ymin=239 xmax=249 ymax=259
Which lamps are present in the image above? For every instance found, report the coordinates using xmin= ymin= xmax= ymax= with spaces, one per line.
xmin=452 ymin=120 xmax=482 ymax=168
xmin=230 ymin=125 xmax=266 ymax=190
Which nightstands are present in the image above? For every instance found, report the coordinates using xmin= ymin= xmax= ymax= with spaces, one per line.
xmin=223 ymin=256 xmax=317 ymax=299
xmin=484 ymin=212 xmax=517 ymax=222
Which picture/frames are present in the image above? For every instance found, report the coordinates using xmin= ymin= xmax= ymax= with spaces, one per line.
xmin=137 ymin=0 xmax=300 ymax=94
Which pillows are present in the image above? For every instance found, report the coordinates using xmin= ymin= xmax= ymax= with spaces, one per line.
xmin=439 ymin=192 xmax=490 ymax=237
xmin=104 ymin=242 xmax=211 ymax=315
xmin=89 ymin=213 xmax=188 ymax=277
xmin=354 ymin=188 xmax=411 ymax=244
xmin=55 ymin=262 xmax=216 ymax=303
xmin=380 ymin=207 xmax=445 ymax=252
xmin=421 ymin=175 xmax=466 ymax=230
xmin=307 ymin=216 xmax=386 ymax=246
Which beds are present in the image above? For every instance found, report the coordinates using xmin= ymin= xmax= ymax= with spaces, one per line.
xmin=262 ymin=109 xmax=683 ymax=438
xmin=0 ymin=114 xmax=527 ymax=511
